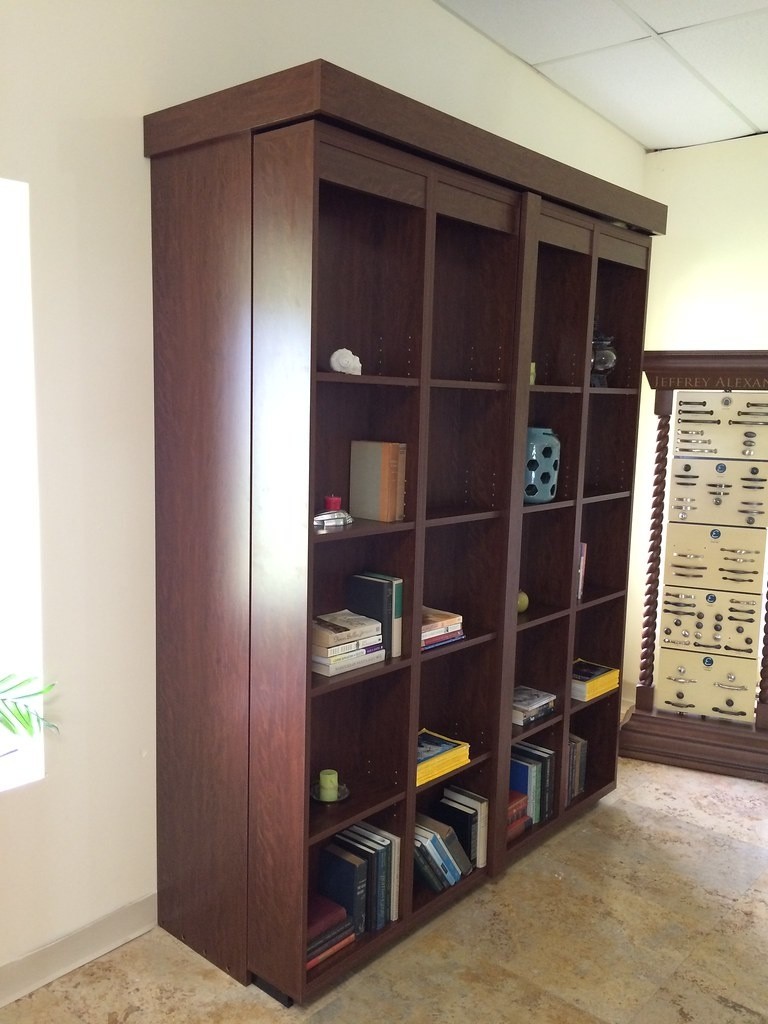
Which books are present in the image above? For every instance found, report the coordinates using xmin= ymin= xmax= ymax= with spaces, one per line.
xmin=312 ymin=572 xmax=403 ymax=679
xmin=350 ymin=439 xmax=408 ymax=521
xmin=414 ymin=785 xmax=489 ymax=891
xmin=507 ymin=740 xmax=556 ymax=842
xmin=577 ymin=542 xmax=587 ymax=600
xmin=307 ymin=821 xmax=401 ymax=971
xmin=416 ymin=727 xmax=471 ymax=786
xmin=568 ymin=731 xmax=587 ymax=803
xmin=421 ymin=604 xmax=465 ymax=651
xmin=512 ymin=684 xmax=556 ymax=727
xmin=571 ymin=658 xmax=620 ymax=702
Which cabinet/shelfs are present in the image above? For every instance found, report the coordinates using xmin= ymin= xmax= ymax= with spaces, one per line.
xmin=142 ymin=53 xmax=670 ymax=1010
